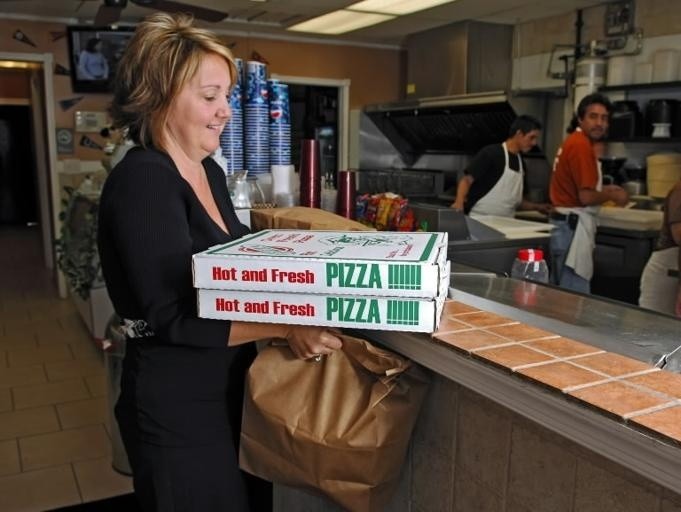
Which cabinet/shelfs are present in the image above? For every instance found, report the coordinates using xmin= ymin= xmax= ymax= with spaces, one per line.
xmin=593 ymin=79 xmax=680 ymax=145
xmin=55 ymin=179 xmax=117 ymax=352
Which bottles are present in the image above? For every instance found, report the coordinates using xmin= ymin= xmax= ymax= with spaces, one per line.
xmin=512 ymin=247 xmax=550 ymax=282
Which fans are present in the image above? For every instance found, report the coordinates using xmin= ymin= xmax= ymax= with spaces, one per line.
xmin=92 ymin=0 xmax=234 ymax=31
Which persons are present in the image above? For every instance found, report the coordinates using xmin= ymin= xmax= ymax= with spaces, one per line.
xmin=449 ymin=115 xmax=552 ymax=218
xmin=79 ymin=37 xmax=109 ymax=81
xmin=638 ymin=183 xmax=681 ymax=318
xmin=97 ymin=11 xmax=343 ymax=512
xmin=548 ymin=94 xmax=630 ymax=296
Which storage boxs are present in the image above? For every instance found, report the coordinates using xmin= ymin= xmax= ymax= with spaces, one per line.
xmin=188 ymin=223 xmax=450 ymax=302
xmin=194 ymin=258 xmax=456 ymax=336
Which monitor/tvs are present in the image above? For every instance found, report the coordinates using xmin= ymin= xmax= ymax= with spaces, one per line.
xmin=66 ymin=24 xmax=135 ymax=93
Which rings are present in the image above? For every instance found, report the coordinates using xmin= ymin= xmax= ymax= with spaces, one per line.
xmin=311 ymin=354 xmax=322 ymax=363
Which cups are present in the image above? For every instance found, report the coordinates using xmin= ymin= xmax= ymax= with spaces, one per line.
xmin=335 ymin=169 xmax=358 ymax=223
xmin=302 ymin=139 xmax=322 ymax=209
xmin=220 ymin=58 xmax=294 ymax=173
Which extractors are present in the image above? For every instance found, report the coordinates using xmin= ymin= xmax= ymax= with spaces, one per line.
xmin=363 ymin=19 xmax=550 ymax=164
